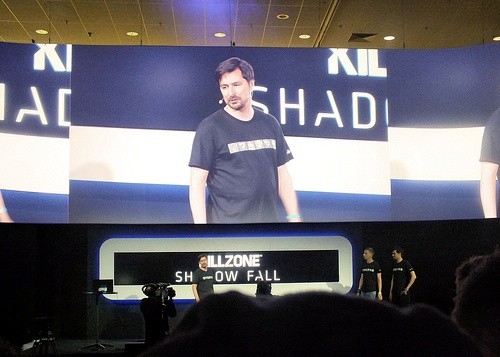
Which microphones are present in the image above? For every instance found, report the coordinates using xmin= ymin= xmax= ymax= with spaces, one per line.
xmin=219 ymin=98 xmax=223 ymax=104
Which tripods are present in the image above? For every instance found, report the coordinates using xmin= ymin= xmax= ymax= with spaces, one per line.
xmin=78 ymin=295 xmax=114 ymax=350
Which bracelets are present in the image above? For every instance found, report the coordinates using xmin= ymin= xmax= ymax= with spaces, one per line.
xmin=405 ymin=288 xmax=409 ymax=291
xmin=285 ymin=214 xmax=303 ymax=219
xmin=378 ymin=293 xmax=382 ymax=294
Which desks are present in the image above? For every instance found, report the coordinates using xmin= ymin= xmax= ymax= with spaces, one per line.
xmin=81 ymin=291 xmax=118 ymax=349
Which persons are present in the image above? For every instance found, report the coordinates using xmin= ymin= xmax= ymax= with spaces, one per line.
xmin=140 ymin=245 xmax=500 ymax=357
xmin=187 ymin=57 xmax=303 ymax=224
xmin=479 ymin=108 xmax=500 ymax=218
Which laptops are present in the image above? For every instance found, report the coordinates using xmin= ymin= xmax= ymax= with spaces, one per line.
xmin=92 ymin=279 xmax=113 ymax=292
